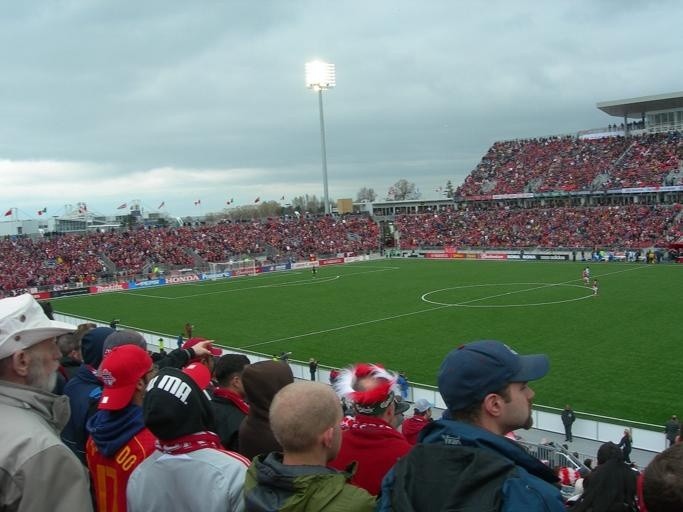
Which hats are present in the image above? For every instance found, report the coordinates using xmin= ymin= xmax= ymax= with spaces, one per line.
xmin=438 ymin=339 xmax=548 ymax=412
xmin=81 ymin=327 xmax=153 ymax=412
xmin=179 ymin=338 xmax=223 ymax=390
xmin=0 ymin=293 xmax=77 ymax=361
xmin=395 ymin=398 xmax=434 ymax=417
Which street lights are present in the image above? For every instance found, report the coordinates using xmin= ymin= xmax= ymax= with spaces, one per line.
xmin=304 ymin=58 xmax=337 ymax=214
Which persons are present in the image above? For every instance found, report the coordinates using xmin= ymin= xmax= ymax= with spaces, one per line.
xmin=1 ymin=120 xmax=683 ymax=512
